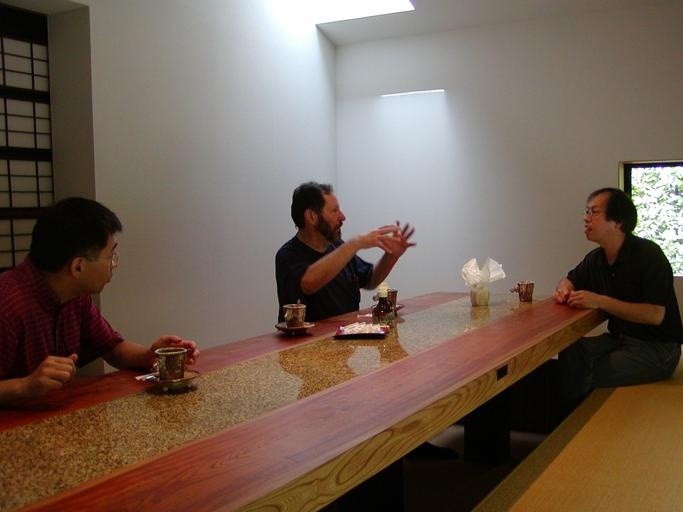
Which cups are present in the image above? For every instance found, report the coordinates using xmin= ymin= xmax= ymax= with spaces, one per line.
xmin=387 ymin=289 xmax=397 ymax=307
xmin=155 ymin=347 xmax=186 ymax=381
xmin=284 ymin=304 xmax=306 ymax=327
xmin=518 ymin=280 xmax=533 ymax=301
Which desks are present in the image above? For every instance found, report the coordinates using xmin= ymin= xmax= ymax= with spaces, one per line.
xmin=2 ymin=291 xmax=611 ymax=509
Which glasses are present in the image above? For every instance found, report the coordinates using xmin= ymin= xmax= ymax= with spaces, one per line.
xmin=89 ymin=252 xmax=120 ymax=267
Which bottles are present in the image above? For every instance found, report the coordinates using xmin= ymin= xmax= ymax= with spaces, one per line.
xmin=372 ymin=298 xmax=392 ymax=324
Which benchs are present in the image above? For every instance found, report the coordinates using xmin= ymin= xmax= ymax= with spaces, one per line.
xmin=472 ymin=352 xmax=683 ymax=511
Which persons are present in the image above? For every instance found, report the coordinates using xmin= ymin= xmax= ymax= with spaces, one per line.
xmin=551 ymin=185 xmax=683 ymax=433
xmin=0 ymin=195 xmax=200 ymax=401
xmin=273 ymin=182 xmax=418 ymax=327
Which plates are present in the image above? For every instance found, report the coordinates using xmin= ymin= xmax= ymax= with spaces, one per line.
xmin=333 ymin=324 xmax=390 ymax=340
xmin=371 ymin=304 xmax=406 ymax=311
xmin=275 ymin=322 xmax=315 ymax=332
xmin=146 ymin=372 xmax=202 ymax=390
